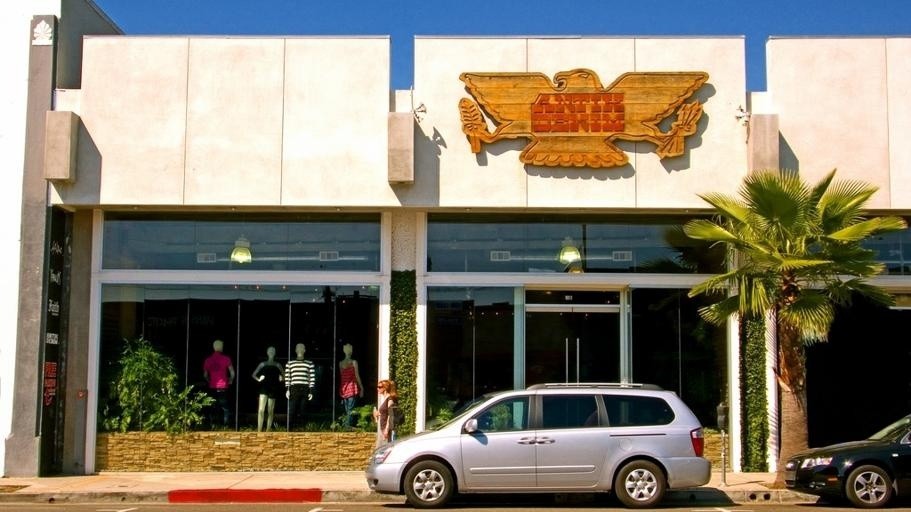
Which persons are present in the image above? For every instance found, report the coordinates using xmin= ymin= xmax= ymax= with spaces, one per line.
xmin=284 ymin=343 xmax=316 ymax=431
xmin=203 ymin=339 xmax=235 ymax=431
xmin=252 ymin=348 xmax=285 ymax=433
xmin=372 ymin=378 xmax=399 ymax=445
xmin=339 ymin=343 xmax=365 ymax=431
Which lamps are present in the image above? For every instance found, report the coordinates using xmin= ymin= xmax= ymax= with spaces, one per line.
xmin=230 ymin=240 xmax=252 ymax=264
xmin=559 ymin=239 xmax=582 ymax=263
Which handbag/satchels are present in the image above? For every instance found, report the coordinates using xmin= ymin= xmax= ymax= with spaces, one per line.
xmin=394 ymin=403 xmax=405 ymax=425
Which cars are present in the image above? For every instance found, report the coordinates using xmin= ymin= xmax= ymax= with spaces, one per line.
xmin=785 ymin=412 xmax=910 ymax=507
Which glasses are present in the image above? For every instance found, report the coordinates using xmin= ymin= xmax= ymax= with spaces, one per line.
xmin=377 ymin=386 xmax=383 ymax=388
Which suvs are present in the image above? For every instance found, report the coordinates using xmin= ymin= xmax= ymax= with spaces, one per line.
xmin=368 ymin=382 xmax=710 ymax=506
